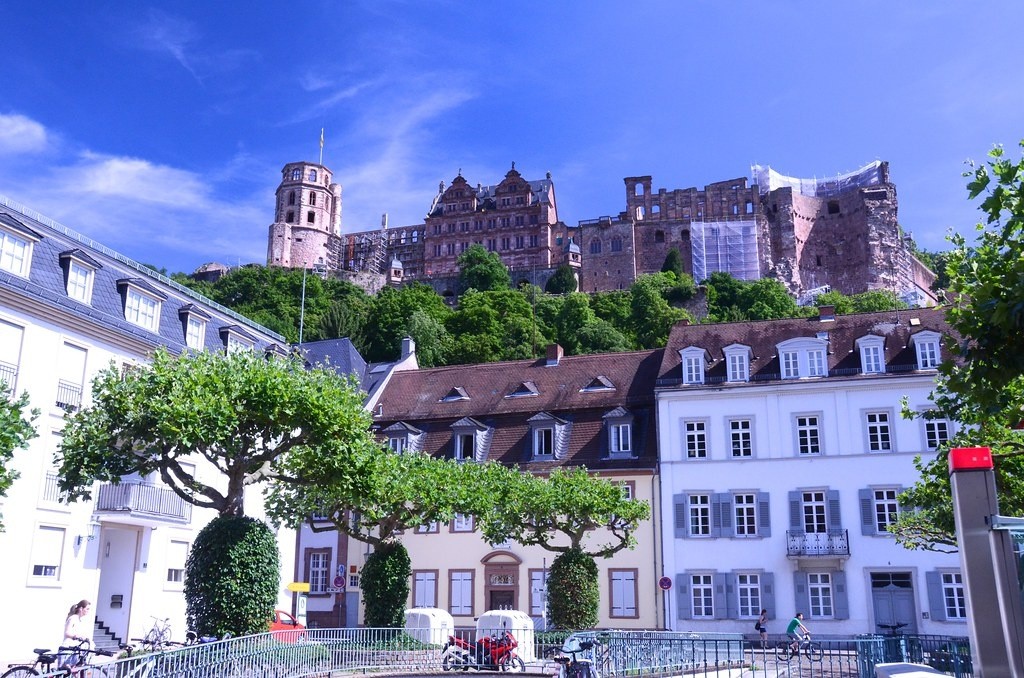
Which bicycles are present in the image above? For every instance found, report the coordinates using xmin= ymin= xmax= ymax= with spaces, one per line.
xmin=0 ymin=632 xmax=278 ymax=678
xmin=542 ymin=640 xmax=600 ymax=678
xmin=142 ymin=616 xmax=172 ymax=650
xmin=774 ymin=631 xmax=825 ymax=662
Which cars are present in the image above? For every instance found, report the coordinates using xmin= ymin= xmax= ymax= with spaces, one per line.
xmin=265 ymin=609 xmax=308 ymax=644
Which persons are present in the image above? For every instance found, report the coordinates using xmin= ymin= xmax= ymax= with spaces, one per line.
xmin=759 ymin=609 xmax=769 ymax=649
xmin=787 ymin=612 xmax=811 ymax=650
xmin=57 ymin=600 xmax=92 ymax=678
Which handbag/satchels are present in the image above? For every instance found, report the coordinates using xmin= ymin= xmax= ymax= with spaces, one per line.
xmin=755 ymin=616 xmax=765 ymax=631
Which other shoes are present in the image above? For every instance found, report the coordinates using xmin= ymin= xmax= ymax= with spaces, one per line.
xmin=790 ymin=645 xmax=796 ymax=652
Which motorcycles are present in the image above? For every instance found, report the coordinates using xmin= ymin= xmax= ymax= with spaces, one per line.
xmin=442 ymin=621 xmax=526 ymax=673
